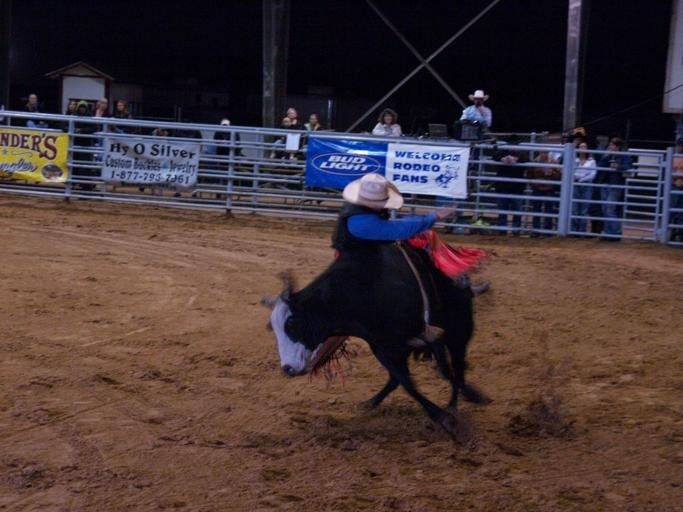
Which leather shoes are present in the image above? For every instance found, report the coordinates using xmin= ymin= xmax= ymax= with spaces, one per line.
xmin=408 ymin=324 xmax=445 ymax=347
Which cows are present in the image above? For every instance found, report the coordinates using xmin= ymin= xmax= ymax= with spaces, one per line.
xmin=260 ymin=240 xmax=494 ymax=441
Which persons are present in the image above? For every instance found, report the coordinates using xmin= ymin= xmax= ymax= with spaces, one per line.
xmin=460 ymin=90 xmax=493 ymax=169
xmin=330 ymin=172 xmax=457 ymax=349
xmin=372 ymin=108 xmax=403 ymax=138
xmin=670 ymin=137 xmax=683 ymax=242
xmin=214 ymin=119 xmax=241 ymax=200
xmin=491 ymin=134 xmax=632 ymax=242
xmin=24 ymin=93 xmax=169 ymax=193
xmin=271 ymin=106 xmax=322 ymax=190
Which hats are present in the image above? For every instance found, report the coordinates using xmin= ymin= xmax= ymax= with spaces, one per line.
xmin=341 ymin=173 xmax=404 ymax=210
xmin=468 ymin=89 xmax=489 ymax=101
xmin=220 ymin=118 xmax=230 ymax=125
